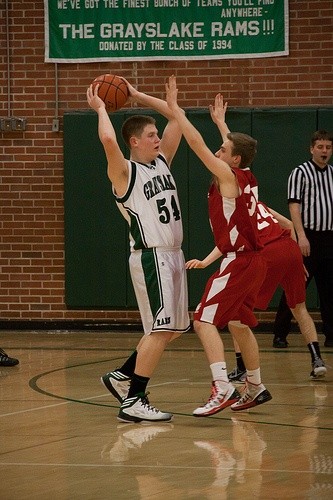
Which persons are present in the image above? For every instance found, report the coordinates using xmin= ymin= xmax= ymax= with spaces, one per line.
xmin=0 ymin=348 xmax=19 ymax=367
xmin=273 ymin=130 xmax=333 ymax=350
xmin=167 ymin=73 xmax=273 ymax=416
xmin=86 ymin=76 xmax=191 ymax=423
xmin=185 ymin=201 xmax=327 ymax=379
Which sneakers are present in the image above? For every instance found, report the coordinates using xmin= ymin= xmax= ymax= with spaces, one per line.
xmin=117 ymin=391 xmax=174 ymax=424
xmin=309 ymin=359 xmax=327 ymax=381
xmin=231 ymin=380 xmax=273 ymax=411
xmin=227 ymin=368 xmax=248 ymax=384
xmin=100 ymin=368 xmax=150 ymax=405
xmin=192 ymin=381 xmax=241 ymax=417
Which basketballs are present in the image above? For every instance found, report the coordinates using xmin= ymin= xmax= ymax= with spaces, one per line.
xmin=90 ymin=71 xmax=131 ymax=111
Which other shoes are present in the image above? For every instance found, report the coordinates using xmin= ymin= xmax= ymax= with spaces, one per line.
xmin=272 ymin=336 xmax=288 ymax=348
xmin=322 ymin=336 xmax=332 ymax=347
xmin=0 ymin=348 xmax=19 ymax=367
